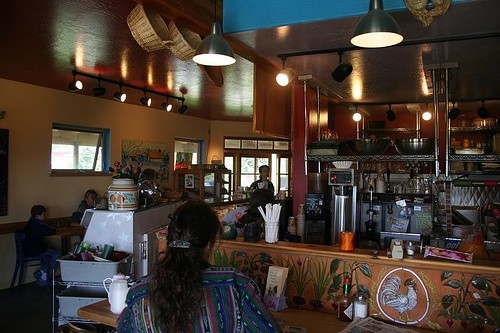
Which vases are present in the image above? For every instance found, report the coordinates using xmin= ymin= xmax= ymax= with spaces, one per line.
xmin=107 ymin=178 xmax=140 ymax=211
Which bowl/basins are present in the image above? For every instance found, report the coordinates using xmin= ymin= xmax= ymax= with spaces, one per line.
xmin=111 ymin=252 xmax=126 ymax=262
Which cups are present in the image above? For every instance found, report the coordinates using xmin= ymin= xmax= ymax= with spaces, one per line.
xmin=244 ymin=224 xmax=260 ymax=242
xmin=287 ymin=204 xmax=306 ymax=242
xmin=222 ymin=224 xmax=237 ymax=240
xmin=81 ymin=252 xmax=88 ymax=260
xmin=264 ymin=222 xmax=279 ymax=243
xmin=375 ymin=168 xmax=386 ymax=194
xmin=102 ymin=244 xmax=114 ymax=259
xmin=339 ymin=231 xmax=355 ymax=251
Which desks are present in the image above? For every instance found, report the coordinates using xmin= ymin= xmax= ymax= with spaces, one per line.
xmin=45 ymin=225 xmax=85 ymax=281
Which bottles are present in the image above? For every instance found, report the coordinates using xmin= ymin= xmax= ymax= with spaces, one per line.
xmin=392 ymin=241 xmax=404 ymax=259
xmin=305 ymin=193 xmax=324 ymax=221
xmin=337 ymin=278 xmax=351 ymax=322
xmin=352 ymin=294 xmax=368 ymax=320
xmin=407 ymin=240 xmax=415 ymax=256
xmin=107 ymin=179 xmax=138 ymax=211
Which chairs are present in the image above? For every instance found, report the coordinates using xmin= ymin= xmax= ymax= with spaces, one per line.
xmin=11 ymin=232 xmax=50 ymax=288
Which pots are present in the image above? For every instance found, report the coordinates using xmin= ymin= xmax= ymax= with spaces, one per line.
xmin=348 ymin=137 xmax=434 ymax=155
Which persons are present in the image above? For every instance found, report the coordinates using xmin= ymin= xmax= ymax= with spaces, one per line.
xmin=115 ymin=199 xmax=283 ymax=333
xmin=22 ymin=205 xmax=59 ymax=287
xmin=78 ymin=189 xmax=98 ymax=216
xmin=248 ymin=165 xmax=274 ymax=198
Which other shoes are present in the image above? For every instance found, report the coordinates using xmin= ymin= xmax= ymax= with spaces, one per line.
xmin=35 ymin=280 xmax=54 ymax=287
xmin=32 ymin=269 xmax=42 ymax=280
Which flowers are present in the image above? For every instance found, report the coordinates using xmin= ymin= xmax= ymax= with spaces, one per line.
xmin=108 ymin=158 xmax=143 ymax=179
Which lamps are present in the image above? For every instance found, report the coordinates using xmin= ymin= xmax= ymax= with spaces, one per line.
xmin=331 ymin=51 xmax=353 ymax=83
xmin=385 ymin=104 xmax=394 ymax=121
xmin=276 ymin=55 xmax=295 ymax=86
xmin=478 ymin=98 xmax=489 ymax=120
xmin=69 ymin=69 xmax=188 ymax=114
xmin=192 ymin=0 xmax=236 ymax=66
xmin=448 ymin=100 xmax=461 ymax=120
xmin=350 ymin=0 xmax=404 ymax=48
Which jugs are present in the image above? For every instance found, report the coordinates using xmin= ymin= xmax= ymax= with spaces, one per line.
xmin=110 ymin=280 xmax=132 ymax=315
xmin=103 ymin=272 xmax=130 ymax=304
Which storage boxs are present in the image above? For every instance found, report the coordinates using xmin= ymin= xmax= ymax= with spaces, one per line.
xmin=56 ymin=249 xmax=135 ymax=327
